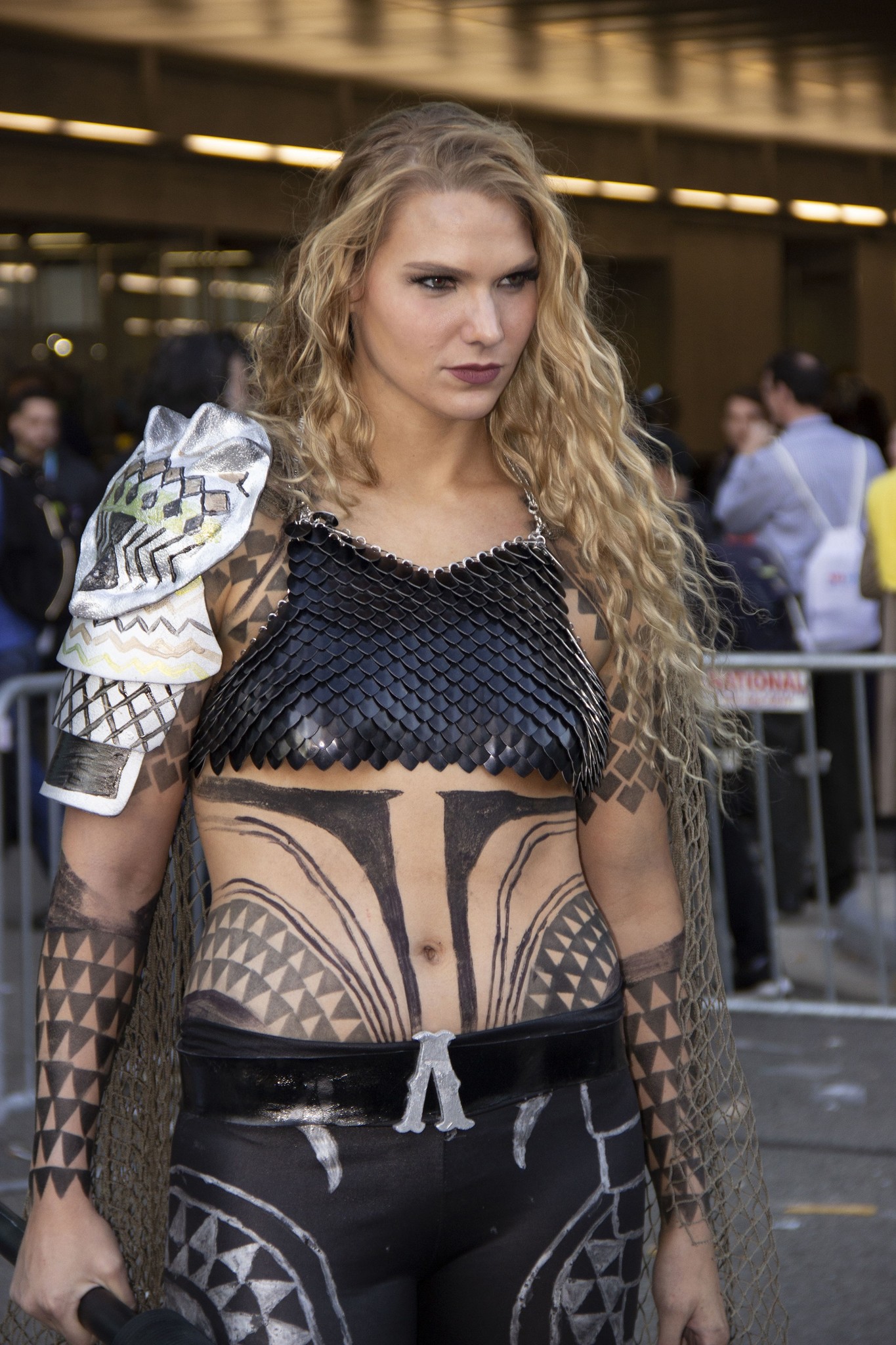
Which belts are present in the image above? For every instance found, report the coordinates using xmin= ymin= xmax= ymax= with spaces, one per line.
xmin=168 ymin=1017 xmax=630 ymax=1129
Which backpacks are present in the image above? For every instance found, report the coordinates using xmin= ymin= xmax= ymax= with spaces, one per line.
xmin=774 ymin=430 xmax=884 ymax=657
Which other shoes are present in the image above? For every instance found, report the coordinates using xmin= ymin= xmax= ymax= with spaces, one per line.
xmin=32 ymin=904 xmax=50 ymax=931
xmin=735 ymin=975 xmax=797 ymax=1002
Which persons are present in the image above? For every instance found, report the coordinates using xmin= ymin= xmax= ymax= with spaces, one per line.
xmin=10 ymin=102 xmax=787 ymax=1345
xmin=649 ymin=346 xmax=896 ymax=1000
xmin=0 ymin=329 xmax=283 ymax=928
